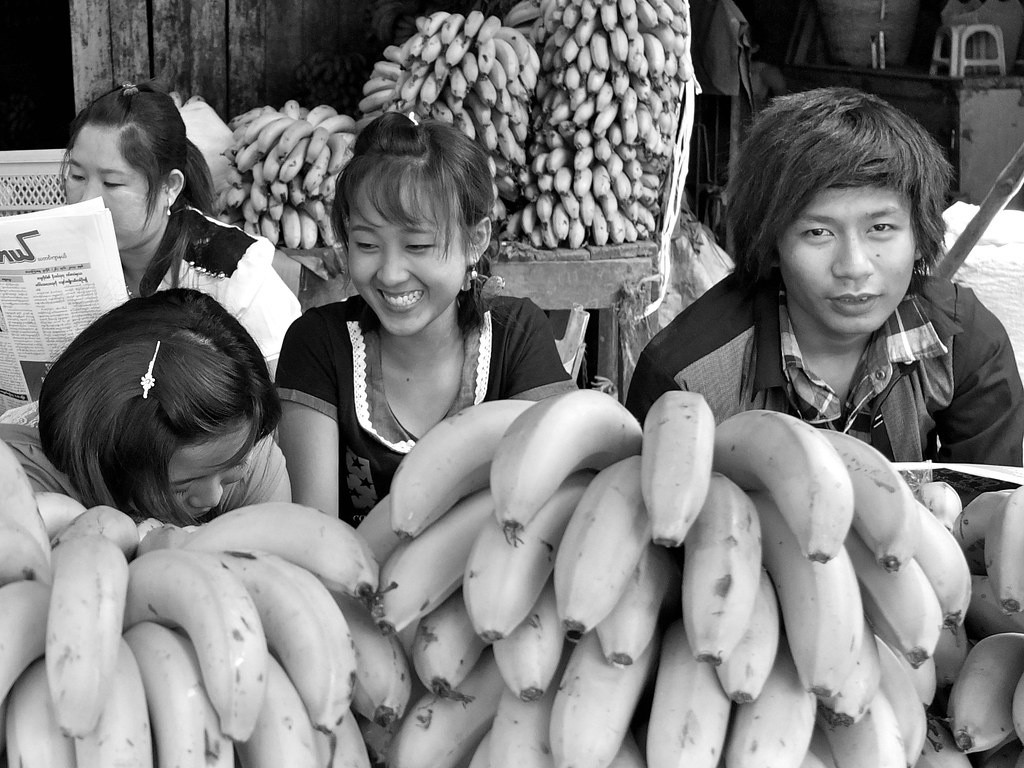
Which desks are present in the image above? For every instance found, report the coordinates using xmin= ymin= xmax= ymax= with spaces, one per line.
xmin=783 ymin=62 xmax=1024 ymax=215
xmin=273 ymin=237 xmax=657 ymax=408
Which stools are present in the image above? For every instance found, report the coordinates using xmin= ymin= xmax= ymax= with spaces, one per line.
xmin=930 ymin=23 xmax=1006 ymax=78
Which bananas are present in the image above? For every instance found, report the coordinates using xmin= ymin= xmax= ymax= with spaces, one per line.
xmin=0 ymin=436 xmax=410 ymax=768
xmin=343 ymin=391 xmax=972 ymax=768
xmin=222 ymin=0 xmax=691 ymax=252
xmin=933 ymin=486 xmax=1024 ymax=768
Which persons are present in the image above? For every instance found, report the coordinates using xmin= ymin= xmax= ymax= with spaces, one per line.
xmin=273 ymin=109 xmax=582 ymax=529
xmin=623 ymin=87 xmax=1024 ymax=467
xmin=58 ymin=82 xmax=301 ymax=385
xmin=0 ymin=288 xmax=291 ymax=527
